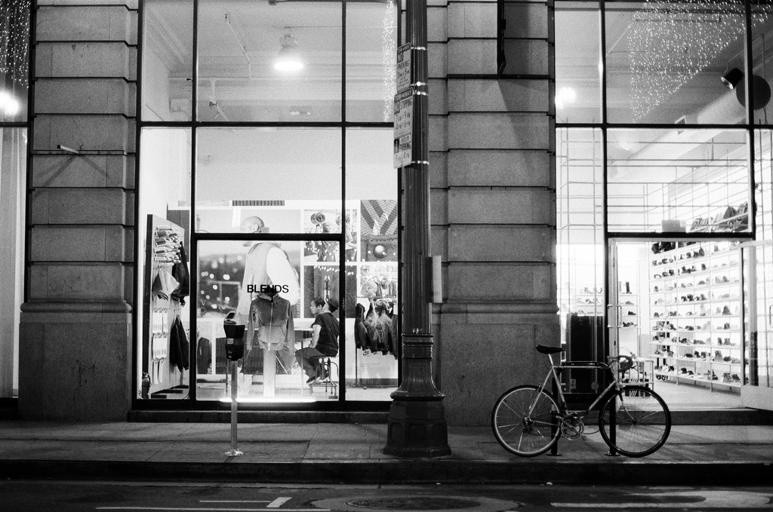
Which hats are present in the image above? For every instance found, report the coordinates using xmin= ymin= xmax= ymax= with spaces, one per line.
xmin=328 ymin=297 xmax=338 ymax=308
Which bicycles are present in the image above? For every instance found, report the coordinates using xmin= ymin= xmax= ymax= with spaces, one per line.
xmin=490 ymin=343 xmax=672 ymax=458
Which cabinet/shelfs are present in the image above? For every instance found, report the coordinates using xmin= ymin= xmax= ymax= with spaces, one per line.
xmin=576 ymin=294 xmax=639 ymax=329
xmin=650 ymin=229 xmax=744 ymax=394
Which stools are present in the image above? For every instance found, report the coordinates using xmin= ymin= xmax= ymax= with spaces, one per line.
xmin=309 ymin=357 xmax=339 ymax=399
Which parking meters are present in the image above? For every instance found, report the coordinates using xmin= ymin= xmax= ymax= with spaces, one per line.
xmin=223 ymin=311 xmax=245 ymax=456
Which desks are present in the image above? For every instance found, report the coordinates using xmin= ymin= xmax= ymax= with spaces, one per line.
xmin=620 ymin=357 xmax=654 ymax=396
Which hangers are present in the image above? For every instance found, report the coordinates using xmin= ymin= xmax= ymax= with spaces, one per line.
xmin=176 ymin=313 xmax=181 ymax=323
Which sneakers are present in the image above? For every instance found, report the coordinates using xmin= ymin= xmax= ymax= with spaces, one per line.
xmin=306 ymin=374 xmax=329 ymax=383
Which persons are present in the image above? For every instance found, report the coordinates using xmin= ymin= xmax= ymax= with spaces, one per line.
xmin=310 ymin=297 xmax=325 ymax=316
xmin=310 ymin=213 xmax=354 ymax=261
xmin=236 ymin=217 xmax=299 ymax=327
xmin=295 ymin=296 xmax=338 ymax=384
